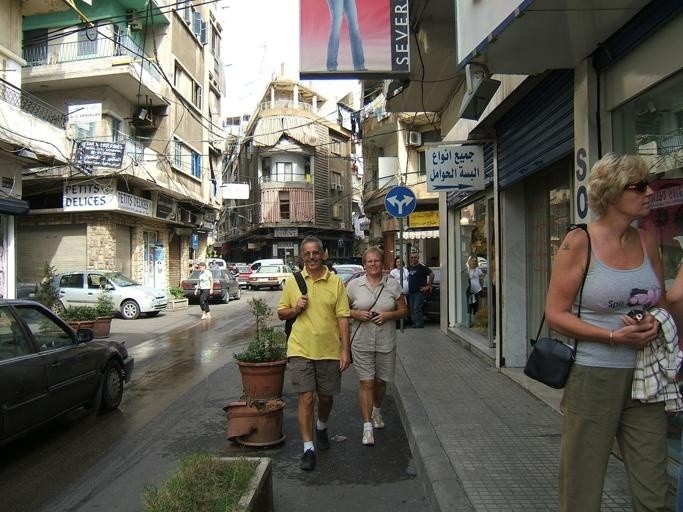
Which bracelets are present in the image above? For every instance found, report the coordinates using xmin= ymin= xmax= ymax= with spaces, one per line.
xmin=609 ymin=329 xmax=617 ymax=346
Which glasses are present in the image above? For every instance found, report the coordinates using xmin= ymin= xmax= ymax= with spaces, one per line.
xmin=625 ymin=180 xmax=648 ymax=193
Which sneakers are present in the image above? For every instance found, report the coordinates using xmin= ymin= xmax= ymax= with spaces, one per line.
xmin=362 ymin=428 xmax=375 ymax=445
xmin=371 ymin=413 xmax=385 ymax=428
xmin=397 ymin=325 xmax=425 ymax=329
xmin=300 ymin=449 xmax=316 ymax=470
xmin=315 ymin=424 xmax=330 ymax=452
xmin=201 ymin=315 xmax=211 ymax=319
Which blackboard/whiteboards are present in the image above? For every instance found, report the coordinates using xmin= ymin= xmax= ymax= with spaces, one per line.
xmin=74 ymin=140 xmax=125 ymax=169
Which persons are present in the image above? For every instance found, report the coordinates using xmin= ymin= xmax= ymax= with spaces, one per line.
xmin=345 ymin=246 xmax=408 ymax=448
xmin=232 ymin=263 xmax=240 ymax=282
xmin=194 ymin=261 xmax=214 ymax=320
xmin=544 ymin=150 xmax=670 ymax=511
xmin=406 ymin=252 xmax=435 ymax=328
xmin=665 ymin=260 xmax=683 ymax=512
xmin=276 ymin=233 xmax=353 ymax=471
xmin=468 ymin=255 xmax=483 ymax=316
xmin=389 ymin=256 xmax=410 ymax=322
xmin=209 ymin=259 xmax=220 ymax=268
xmin=326 ymin=0 xmax=367 ymax=72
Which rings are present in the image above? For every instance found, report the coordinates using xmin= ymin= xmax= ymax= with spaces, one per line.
xmin=379 ymin=321 xmax=383 ymax=324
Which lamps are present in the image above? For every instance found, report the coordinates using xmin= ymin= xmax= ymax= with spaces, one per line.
xmin=457 ymin=62 xmax=499 ymax=124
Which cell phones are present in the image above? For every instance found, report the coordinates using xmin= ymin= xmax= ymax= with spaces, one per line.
xmin=370 ymin=312 xmax=379 ymax=318
xmin=627 ymin=310 xmax=646 ymax=322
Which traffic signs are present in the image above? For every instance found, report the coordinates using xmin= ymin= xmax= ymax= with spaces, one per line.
xmin=422 ymin=146 xmax=486 ymax=192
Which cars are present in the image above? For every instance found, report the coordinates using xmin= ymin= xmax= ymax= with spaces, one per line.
xmin=181 ymin=268 xmax=242 ymax=304
xmin=204 ymin=256 xmax=365 ymax=290
xmin=343 ymin=270 xmax=392 ymax=288
xmin=16 ymin=274 xmax=38 ymax=300
xmin=464 ymin=254 xmax=487 ymax=297
xmin=396 ymin=265 xmax=441 ymax=325
xmin=34 ymin=270 xmax=169 ymax=320
xmin=0 ymin=294 xmax=134 ymax=449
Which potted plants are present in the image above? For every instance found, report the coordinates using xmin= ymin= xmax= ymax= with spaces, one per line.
xmin=223 ymin=389 xmax=286 ymax=447
xmin=164 ymin=287 xmax=188 ymax=311
xmin=149 ymin=452 xmax=277 ymax=512
xmin=235 ymin=291 xmax=287 ymax=399
xmin=65 ymin=301 xmax=92 ymax=332
xmin=93 ymin=292 xmax=115 ymax=339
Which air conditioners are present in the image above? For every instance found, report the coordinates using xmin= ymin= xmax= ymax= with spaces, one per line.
xmin=126 ymin=10 xmax=142 ymax=30
xmin=135 ymin=112 xmax=156 ymax=129
xmin=406 ymin=131 xmax=421 ymax=148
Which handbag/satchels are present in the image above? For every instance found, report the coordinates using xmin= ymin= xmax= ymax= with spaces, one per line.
xmin=523 ymin=312 xmax=577 ymax=389
xmin=284 ymin=272 xmax=308 ymax=335
